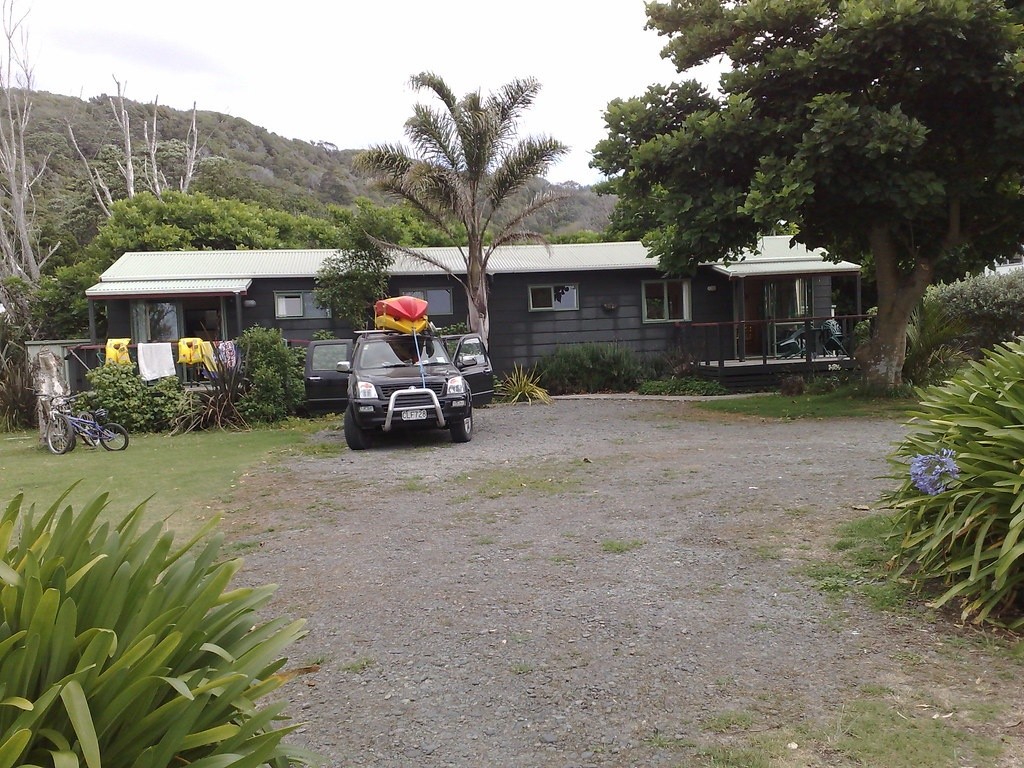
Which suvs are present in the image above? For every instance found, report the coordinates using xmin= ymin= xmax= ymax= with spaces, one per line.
xmin=303 ymin=330 xmax=494 ymax=450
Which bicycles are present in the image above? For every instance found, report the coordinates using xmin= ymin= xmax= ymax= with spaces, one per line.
xmin=24 ymin=387 xmax=130 ymax=454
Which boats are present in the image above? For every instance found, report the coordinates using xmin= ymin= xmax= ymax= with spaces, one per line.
xmin=374 ymin=296 xmax=430 ymax=320
xmin=374 ymin=314 xmax=429 ymax=334
